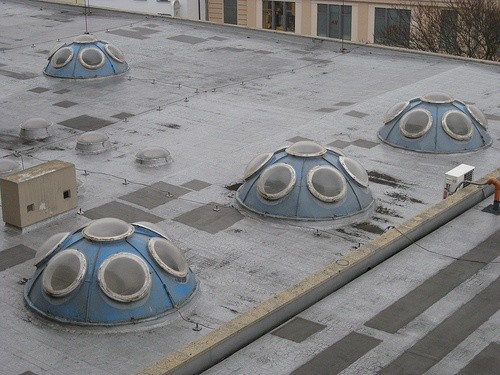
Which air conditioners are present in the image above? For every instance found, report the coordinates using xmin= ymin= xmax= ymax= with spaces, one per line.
xmin=443 ymin=164 xmax=475 ymax=200
